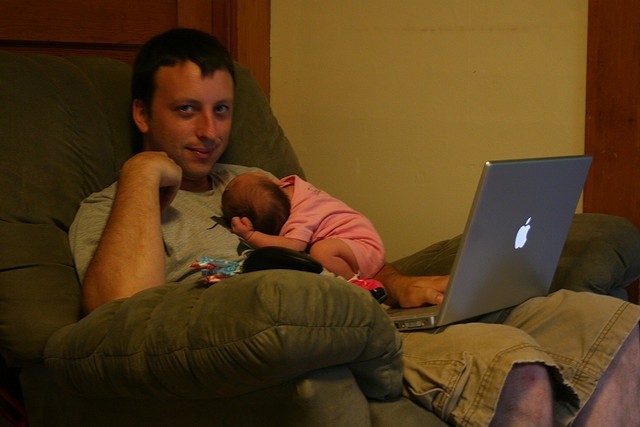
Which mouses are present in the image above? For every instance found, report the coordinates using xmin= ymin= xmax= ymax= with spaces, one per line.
xmin=240 ymin=243 xmax=324 ymax=274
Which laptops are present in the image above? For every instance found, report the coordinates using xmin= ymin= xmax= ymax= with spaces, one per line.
xmin=383 ymin=153 xmax=595 ymax=332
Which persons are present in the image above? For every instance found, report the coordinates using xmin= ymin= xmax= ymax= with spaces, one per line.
xmin=220 ymin=170 xmax=388 ymax=305
